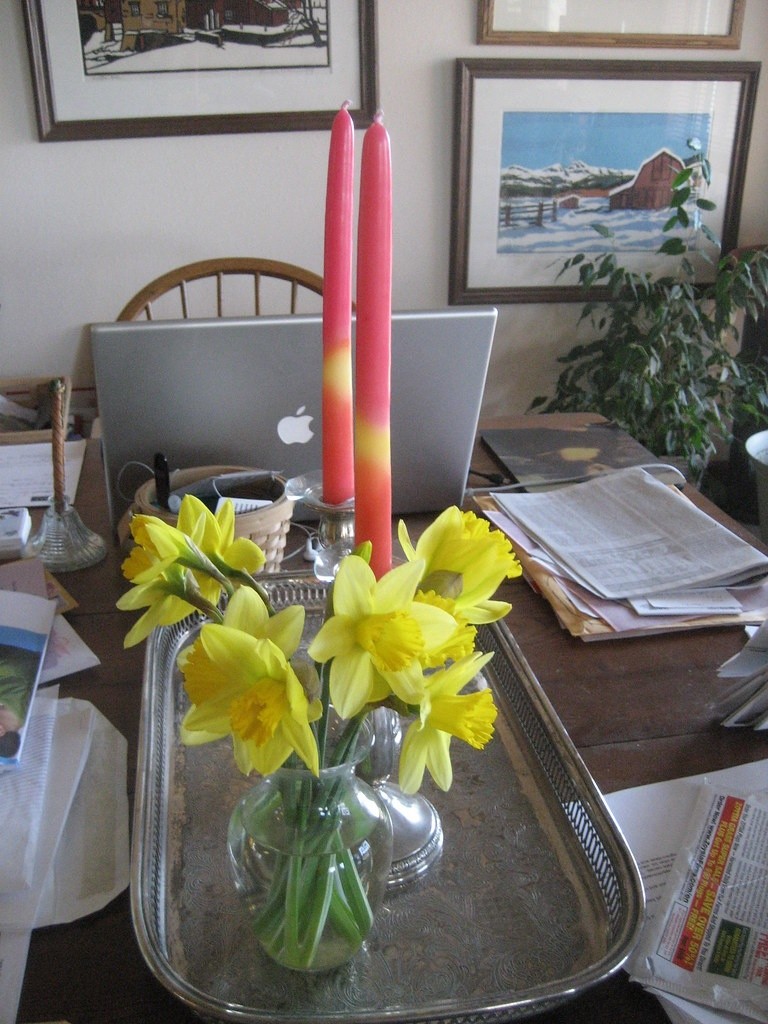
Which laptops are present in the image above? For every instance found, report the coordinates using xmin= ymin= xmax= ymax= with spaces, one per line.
xmin=92 ymin=304 xmax=497 ymax=531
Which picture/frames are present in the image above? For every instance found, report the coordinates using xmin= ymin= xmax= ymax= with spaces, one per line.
xmin=475 ymin=0 xmax=747 ymax=50
xmin=447 ymin=57 xmax=764 ymax=304
xmin=22 ymin=0 xmax=382 ymax=143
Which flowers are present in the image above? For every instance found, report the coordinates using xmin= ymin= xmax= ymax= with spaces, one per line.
xmin=116 ymin=494 xmax=523 ymax=967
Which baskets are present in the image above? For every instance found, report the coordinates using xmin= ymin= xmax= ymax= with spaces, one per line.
xmin=135 ymin=464 xmax=294 ymax=573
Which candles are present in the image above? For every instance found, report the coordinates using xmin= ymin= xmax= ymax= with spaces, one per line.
xmin=356 ymin=110 xmax=394 ymax=578
xmin=321 ymin=100 xmax=357 ymax=506
xmin=49 ymin=379 xmax=65 ymax=513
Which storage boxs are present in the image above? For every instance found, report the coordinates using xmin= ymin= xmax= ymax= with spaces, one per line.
xmin=0 ymin=377 xmax=73 ymax=445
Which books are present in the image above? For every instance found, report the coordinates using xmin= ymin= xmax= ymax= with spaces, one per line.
xmin=0 ymin=586 xmax=58 ymax=766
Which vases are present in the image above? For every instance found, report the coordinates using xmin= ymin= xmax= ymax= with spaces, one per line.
xmin=226 ymin=700 xmax=395 ymax=973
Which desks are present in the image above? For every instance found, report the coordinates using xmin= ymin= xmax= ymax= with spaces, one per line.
xmin=0 ymin=413 xmax=768 ymax=1024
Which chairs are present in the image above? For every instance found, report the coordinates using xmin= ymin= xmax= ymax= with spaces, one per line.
xmin=117 ymin=255 xmax=357 ymax=321
